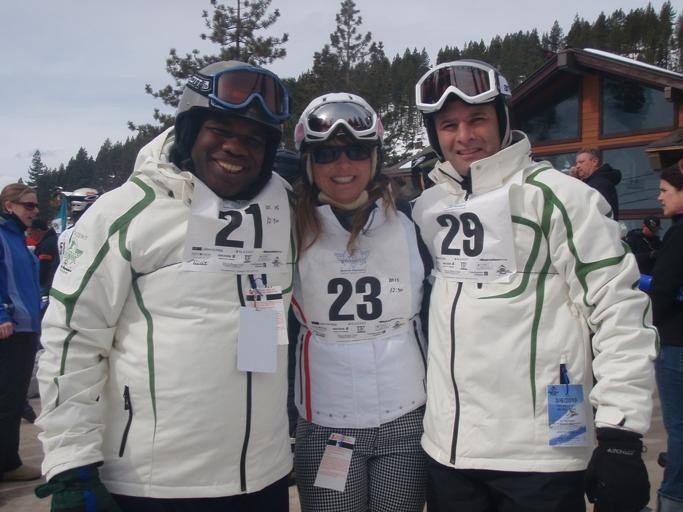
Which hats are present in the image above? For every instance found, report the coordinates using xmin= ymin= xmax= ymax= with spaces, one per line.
xmin=645 ymin=215 xmax=662 ymax=232
xmin=660 ymin=159 xmax=683 ymax=188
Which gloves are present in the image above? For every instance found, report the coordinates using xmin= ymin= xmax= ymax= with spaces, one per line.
xmin=35 ymin=467 xmax=117 ymax=512
xmin=585 ymin=424 xmax=650 ymax=512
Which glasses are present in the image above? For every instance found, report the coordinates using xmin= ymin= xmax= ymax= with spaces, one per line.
xmin=309 ymin=147 xmax=373 ymax=163
xmin=185 ymin=67 xmax=289 ymax=121
xmin=416 ymin=63 xmax=510 ymax=110
xmin=300 ymin=99 xmax=378 ymax=142
xmin=12 ymin=200 xmax=38 ymax=212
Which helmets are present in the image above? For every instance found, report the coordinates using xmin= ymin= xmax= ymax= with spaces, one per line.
xmin=172 ymin=59 xmax=290 ymax=131
xmin=413 ymin=59 xmax=510 ymax=115
xmin=292 ymin=92 xmax=385 ymax=146
xmin=69 ymin=186 xmax=97 ymax=216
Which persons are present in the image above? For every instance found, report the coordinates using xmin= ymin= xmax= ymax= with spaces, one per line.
xmin=282 ymin=92 xmax=437 ymax=511
xmin=627 ymin=161 xmax=682 ymax=511
xmin=0 ymin=182 xmax=102 ymax=512
xmin=572 ymin=146 xmax=622 ymax=222
xmin=407 ymin=58 xmax=663 ymax=511
xmin=34 ymin=61 xmax=300 ymax=511
